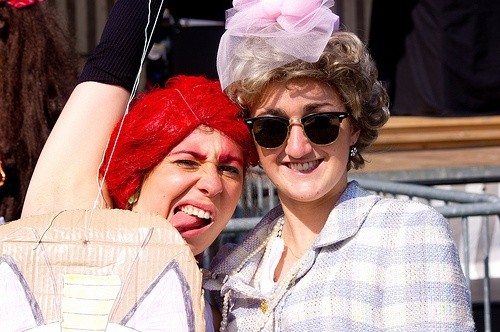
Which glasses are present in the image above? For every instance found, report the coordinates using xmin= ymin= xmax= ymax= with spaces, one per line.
xmin=244 ymin=112 xmax=349 ymax=150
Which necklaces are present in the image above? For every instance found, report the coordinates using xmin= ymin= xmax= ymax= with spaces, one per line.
xmin=285 ymin=244 xmax=301 ymax=261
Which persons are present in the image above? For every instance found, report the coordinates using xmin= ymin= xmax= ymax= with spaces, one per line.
xmin=21 ymin=0 xmax=261 ymax=332
xmin=202 ymin=0 xmax=476 ymax=332
